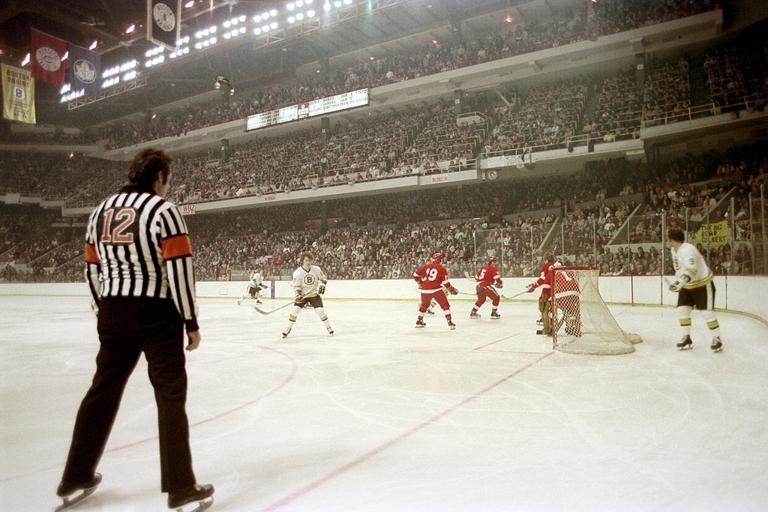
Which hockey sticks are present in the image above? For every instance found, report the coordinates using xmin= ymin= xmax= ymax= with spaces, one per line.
xmin=501 ymin=290 xmax=527 ymax=299
xmin=253 ymin=290 xmax=314 ymax=315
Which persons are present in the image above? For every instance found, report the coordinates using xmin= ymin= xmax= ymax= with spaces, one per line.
xmin=3 ymin=121 xmax=766 ymax=281
xmin=57 ymin=149 xmax=216 ymax=511
xmin=468 ymin=256 xmax=503 ymax=317
xmin=666 ymin=228 xmax=723 ymax=350
xmin=282 ymin=251 xmax=335 ymax=336
xmin=103 ymin=0 xmax=765 ymax=121
xmin=525 ymin=251 xmax=557 ymax=323
xmin=411 ymin=253 xmax=459 ymax=327
xmin=536 ymin=261 xmax=582 ymax=337
xmin=236 ymin=269 xmax=269 ymax=305
xmin=424 ymin=284 xmax=450 ymax=314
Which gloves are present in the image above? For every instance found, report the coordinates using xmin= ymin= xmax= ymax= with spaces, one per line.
xmin=669 ymin=280 xmax=685 ymax=292
xmin=318 ymin=285 xmax=325 ymax=295
xmin=496 ymin=282 xmax=501 ymax=287
xmin=296 ymin=297 xmax=303 ymax=304
xmin=451 ymin=287 xmax=459 ymax=295
xmin=526 ymin=282 xmax=538 ymax=292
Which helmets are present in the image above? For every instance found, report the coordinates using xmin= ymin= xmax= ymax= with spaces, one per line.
xmin=432 ymin=252 xmax=444 ymax=259
xmin=490 ymin=257 xmax=496 ymax=261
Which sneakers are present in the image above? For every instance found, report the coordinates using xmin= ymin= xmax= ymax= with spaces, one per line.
xmin=326 ymin=327 xmax=334 ymax=334
xmin=57 ymin=472 xmax=101 ymax=497
xmin=677 ymin=335 xmax=693 ymax=347
xmin=470 ymin=312 xmax=481 ymax=317
xmin=711 ymin=337 xmax=722 ymax=350
xmin=416 ymin=320 xmax=426 ymax=325
xmin=167 ymin=485 xmax=213 ymax=508
xmin=281 ymin=325 xmax=291 ymax=336
xmin=491 ymin=313 xmax=500 ymax=317
xmin=448 ymin=321 xmax=455 ymax=326
